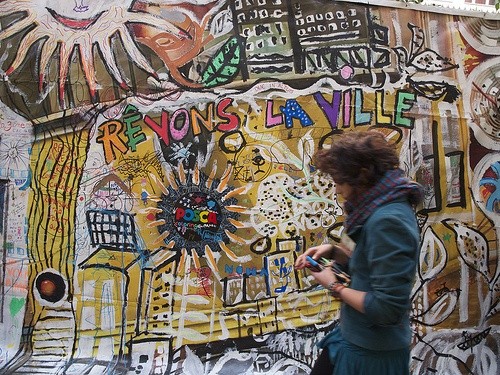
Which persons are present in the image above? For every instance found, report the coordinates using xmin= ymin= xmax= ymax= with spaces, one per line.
xmin=294 ymin=131 xmax=424 ymax=374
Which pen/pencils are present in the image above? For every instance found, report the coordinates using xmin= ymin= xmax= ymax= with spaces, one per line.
xmin=304 ymin=253 xmax=352 ymax=290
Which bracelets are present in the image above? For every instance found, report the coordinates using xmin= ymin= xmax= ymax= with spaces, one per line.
xmin=328 ymin=281 xmax=346 ymax=295
